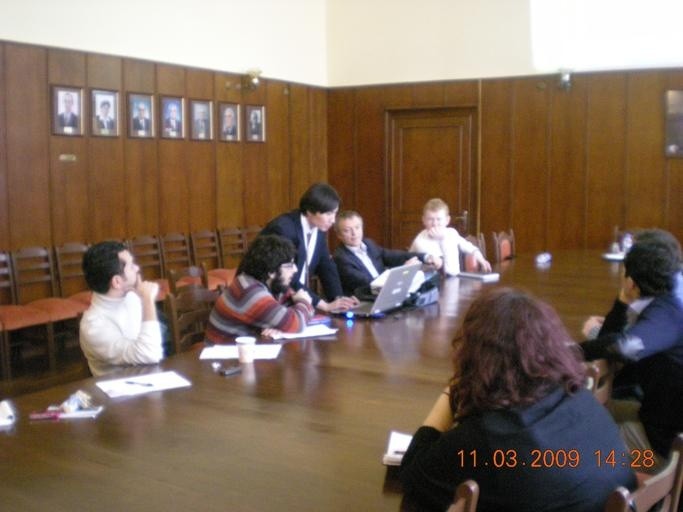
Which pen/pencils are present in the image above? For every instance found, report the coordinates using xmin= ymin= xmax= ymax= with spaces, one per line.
xmin=125 ymin=381 xmax=153 ymax=387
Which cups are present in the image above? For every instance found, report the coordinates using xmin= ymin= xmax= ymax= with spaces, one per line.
xmin=235 ymin=336 xmax=256 ymax=365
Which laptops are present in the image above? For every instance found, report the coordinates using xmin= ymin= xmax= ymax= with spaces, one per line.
xmin=330 ymin=262 xmax=422 ymax=318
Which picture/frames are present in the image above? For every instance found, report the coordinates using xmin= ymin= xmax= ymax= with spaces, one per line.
xmin=158 ymin=93 xmax=186 ymax=140
xmin=662 ymin=88 xmax=683 ymax=159
xmin=125 ymin=90 xmax=157 ymax=140
xmin=88 ymin=87 xmax=121 ymax=138
xmin=49 ymin=84 xmax=85 ymax=137
xmin=243 ymin=103 xmax=267 ymax=143
xmin=187 ymin=97 xmax=215 ymax=142
xmin=215 ymin=100 xmax=243 ymax=142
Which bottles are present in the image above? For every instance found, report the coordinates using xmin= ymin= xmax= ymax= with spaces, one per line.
xmin=624 ymin=234 xmax=632 ymax=257
xmin=535 ymin=252 xmax=552 ymax=264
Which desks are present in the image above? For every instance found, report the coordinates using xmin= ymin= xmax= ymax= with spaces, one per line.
xmin=0 ymin=246 xmax=625 ymax=512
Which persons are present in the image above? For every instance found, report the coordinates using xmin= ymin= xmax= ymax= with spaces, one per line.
xmin=222 ymin=108 xmax=236 ymax=135
xmin=206 ymin=233 xmax=316 ymax=347
xmin=58 ymin=93 xmax=80 ymax=128
xmin=333 ymin=209 xmax=442 ymax=296
xmin=400 ymin=285 xmax=638 ymax=512
xmin=79 ymin=241 xmax=166 ymax=377
xmin=582 ymin=228 xmax=683 ymax=401
xmin=133 ymin=103 xmax=149 ymax=130
xmin=567 ymin=237 xmax=683 ymax=475
xmin=165 ymin=104 xmax=181 ymax=131
xmin=249 ymin=112 xmax=261 ymax=134
xmin=235 ymin=183 xmax=360 ymax=313
xmin=408 ymin=198 xmax=492 ymax=278
xmin=97 ymin=100 xmax=114 ymax=129
xmin=194 ymin=108 xmax=209 ymax=133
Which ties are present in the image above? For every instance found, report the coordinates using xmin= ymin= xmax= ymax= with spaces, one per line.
xmin=307 ymin=233 xmax=311 ymax=246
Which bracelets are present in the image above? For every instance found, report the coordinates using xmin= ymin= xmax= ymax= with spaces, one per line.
xmin=442 ymin=392 xmax=458 ymax=405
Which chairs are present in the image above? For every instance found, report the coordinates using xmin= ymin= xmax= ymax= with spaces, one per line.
xmin=459 ymin=232 xmax=488 ymax=272
xmin=604 ymin=432 xmax=683 ymax=512
xmin=585 ymin=356 xmax=616 ymax=409
xmin=445 ymin=479 xmax=481 ymax=512
xmin=491 ymin=229 xmax=516 ymax=262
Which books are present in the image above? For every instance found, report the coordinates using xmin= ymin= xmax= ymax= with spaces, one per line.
xmin=383 ymin=431 xmax=413 ymax=466
